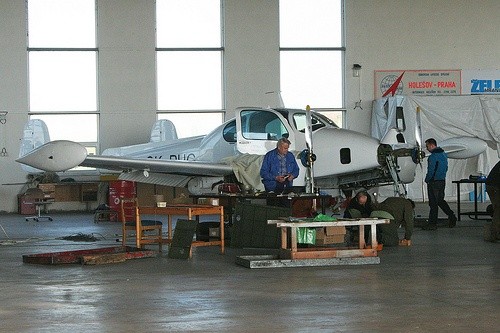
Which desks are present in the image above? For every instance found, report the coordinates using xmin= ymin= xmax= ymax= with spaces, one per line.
xmin=452 ymin=178 xmax=493 ymax=221
xmin=267 ymin=218 xmax=390 ymax=259
xmin=190 ymin=194 xmax=331 ymax=244
xmin=137 ymin=204 xmax=225 ymax=258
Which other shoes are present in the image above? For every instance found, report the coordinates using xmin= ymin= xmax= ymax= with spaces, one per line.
xmin=422 ymin=223 xmax=437 ymax=230
xmin=448 ymin=214 xmax=457 ymax=228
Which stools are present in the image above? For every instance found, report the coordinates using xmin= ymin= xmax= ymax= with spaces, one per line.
xmin=23 ymin=198 xmax=55 ymax=221
xmin=93 ymin=210 xmax=119 ymax=225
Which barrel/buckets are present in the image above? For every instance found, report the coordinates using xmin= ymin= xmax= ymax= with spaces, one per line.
xmin=109 ymin=179 xmax=136 ymax=221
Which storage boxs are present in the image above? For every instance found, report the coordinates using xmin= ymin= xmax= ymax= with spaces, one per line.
xmin=315 ymin=226 xmax=347 ymax=245
xmin=199 ymin=222 xmax=226 ymax=242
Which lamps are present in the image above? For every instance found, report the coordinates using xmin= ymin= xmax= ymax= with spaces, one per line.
xmin=352 ymin=64 xmax=362 ymax=77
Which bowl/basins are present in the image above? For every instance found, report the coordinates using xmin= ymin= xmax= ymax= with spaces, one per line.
xmin=157 ymin=202 xmax=166 ymax=208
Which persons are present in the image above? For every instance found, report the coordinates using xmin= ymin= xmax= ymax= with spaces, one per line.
xmin=261 ymin=138 xmax=299 ymax=194
xmin=485 ymin=161 xmax=500 ymax=243
xmin=373 ymin=197 xmax=416 ymax=247
xmin=422 ymin=138 xmax=457 ymax=230
xmin=344 ymin=190 xmax=378 ymax=247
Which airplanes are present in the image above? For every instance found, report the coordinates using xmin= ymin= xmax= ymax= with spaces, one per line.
xmin=16 ymin=105 xmax=488 ymax=199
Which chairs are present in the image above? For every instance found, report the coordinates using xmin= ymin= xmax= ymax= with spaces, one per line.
xmin=121 ymin=199 xmax=162 ymax=253
